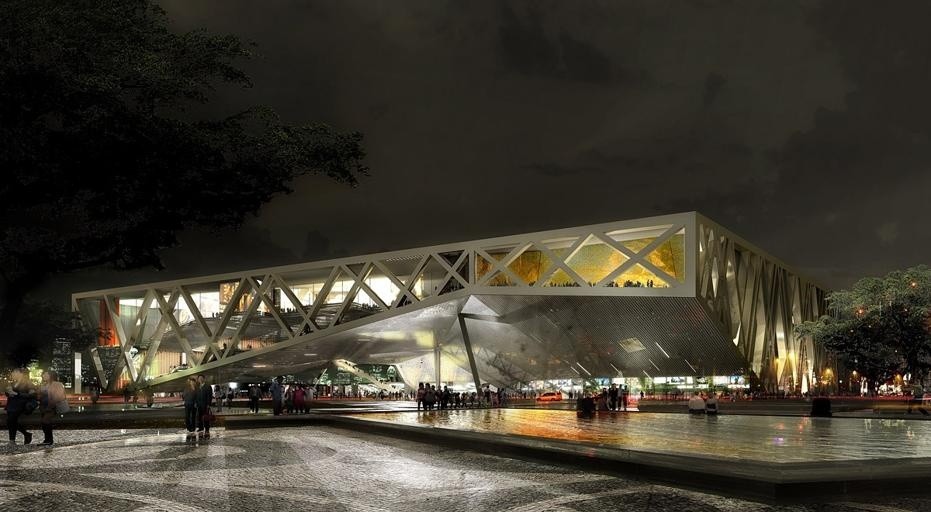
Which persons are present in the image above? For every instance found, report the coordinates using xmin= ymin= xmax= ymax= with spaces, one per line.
xmin=270 ymin=376 xmax=316 ymax=415
xmin=214 ymin=384 xmax=222 ymax=412
xmin=478 ymin=385 xmax=507 ymax=408
xmin=689 ymin=391 xmax=706 ymax=414
xmin=569 ymin=390 xmax=580 ymax=401
xmin=416 ymin=383 xmax=476 ymax=410
xmin=133 ymin=388 xmax=138 ymax=403
xmin=123 ymin=387 xmax=130 ymax=402
xmin=603 ymin=383 xmax=629 ymax=411
xmin=183 ymin=375 xmax=213 ymax=436
xmin=249 ymin=384 xmax=262 ymax=413
xmin=706 ymin=394 xmax=718 ymax=414
xmin=144 ymin=385 xmax=154 ymax=408
xmin=902 ymin=379 xmax=930 ymax=417
xmin=3 ymin=366 xmax=36 ymax=448
xmin=28 ymin=369 xmax=68 ymax=447
xmin=372 ymin=390 xmax=412 ymax=400
xmin=228 ymin=388 xmax=232 ymax=409
xmin=640 ymin=391 xmax=644 ymax=399
xmin=90 ymin=386 xmax=99 ymax=409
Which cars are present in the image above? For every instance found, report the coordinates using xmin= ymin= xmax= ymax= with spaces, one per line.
xmin=536 ymin=391 xmax=562 ymax=402
